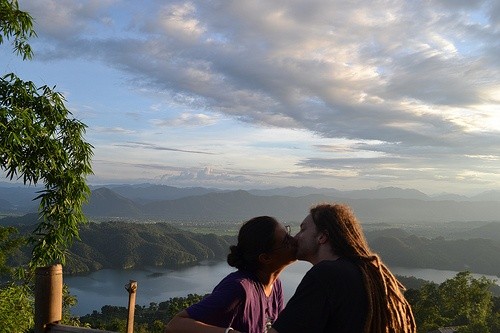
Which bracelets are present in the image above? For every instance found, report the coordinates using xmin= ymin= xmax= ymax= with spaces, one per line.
xmin=225 ymin=327 xmax=234 ymax=333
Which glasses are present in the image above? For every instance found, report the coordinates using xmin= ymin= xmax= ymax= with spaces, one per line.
xmin=260 ymin=224 xmax=291 ymax=256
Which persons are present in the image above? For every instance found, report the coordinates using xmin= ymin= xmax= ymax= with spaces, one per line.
xmin=267 ymin=204 xmax=416 ymax=333
xmin=166 ymin=216 xmax=298 ymax=333
